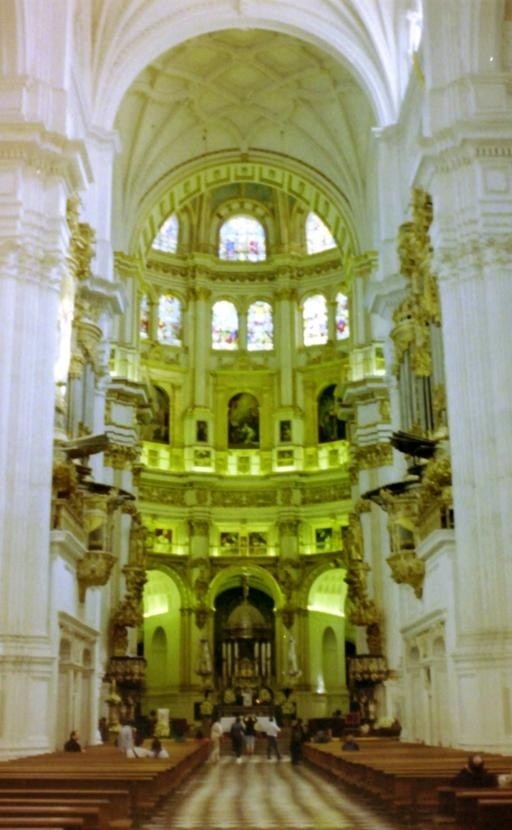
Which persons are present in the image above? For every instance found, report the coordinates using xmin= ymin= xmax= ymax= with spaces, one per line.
xmin=65 ymin=732 xmax=85 ymax=753
xmin=442 ymin=753 xmax=499 ymax=797
xmin=265 ymin=717 xmax=286 ymax=761
xmin=150 ymin=737 xmax=171 ymax=760
xmin=231 ymin=717 xmax=247 ymax=763
xmin=290 ymin=718 xmax=307 ymax=765
xmin=243 ymin=714 xmax=259 ymax=756
xmin=115 ymin=720 xmax=138 ymax=760
xmin=195 ymin=730 xmax=207 ymax=743
xmin=344 ymin=734 xmax=360 ymax=751
xmin=210 ymin=717 xmax=224 ymax=764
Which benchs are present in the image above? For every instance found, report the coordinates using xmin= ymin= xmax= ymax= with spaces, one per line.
xmin=0 ymin=736 xmax=212 ymax=830
xmin=301 ymin=734 xmax=511 ymax=828
